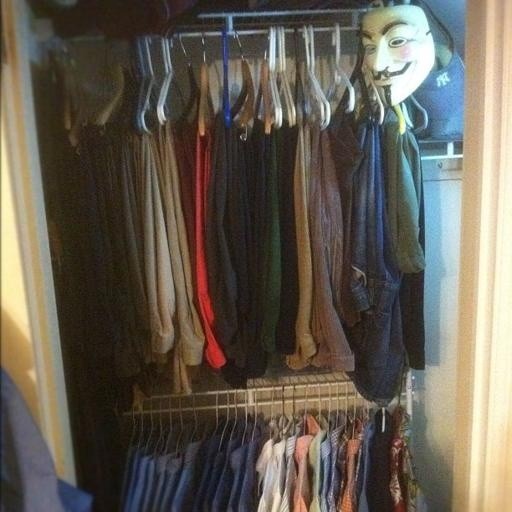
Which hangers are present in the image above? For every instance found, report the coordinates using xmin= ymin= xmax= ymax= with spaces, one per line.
xmin=127 ymin=368 xmax=414 ymax=465
xmin=40 ymin=21 xmax=429 ymax=140
